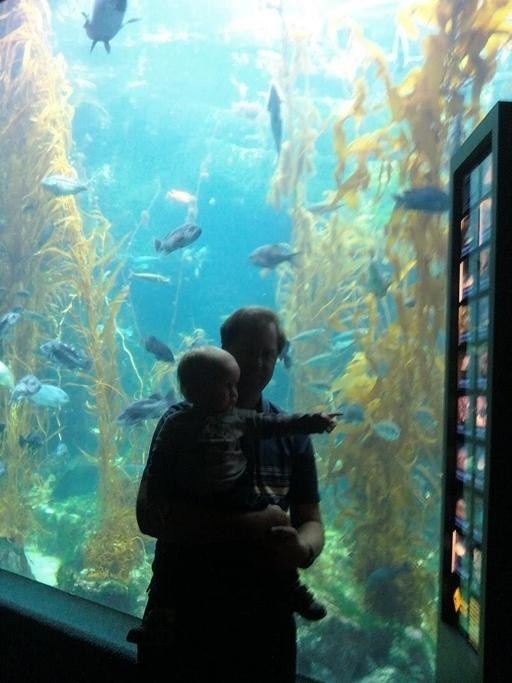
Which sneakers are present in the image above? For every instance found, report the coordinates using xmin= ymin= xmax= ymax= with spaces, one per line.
xmin=124 ymin=623 xmax=173 ymax=648
xmin=284 ymin=584 xmax=327 ymax=621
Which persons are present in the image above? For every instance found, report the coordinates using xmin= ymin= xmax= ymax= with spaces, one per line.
xmin=123 ymin=340 xmax=346 ymax=645
xmin=131 ymin=303 xmax=327 ymax=682
